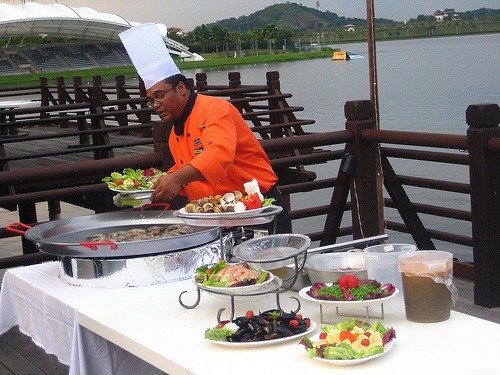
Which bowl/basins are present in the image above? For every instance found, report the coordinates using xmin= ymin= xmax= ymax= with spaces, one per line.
xmin=298 ymin=248 xmax=368 ymax=286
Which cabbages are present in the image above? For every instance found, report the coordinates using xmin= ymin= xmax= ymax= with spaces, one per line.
xmin=300 ymin=320 xmax=390 ymax=360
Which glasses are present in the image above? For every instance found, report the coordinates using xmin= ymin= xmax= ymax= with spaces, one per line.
xmin=145 ymin=82 xmax=178 ymax=110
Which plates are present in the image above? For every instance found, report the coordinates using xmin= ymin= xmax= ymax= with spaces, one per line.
xmin=107 ymin=185 xmax=156 ymax=195
xmin=192 ymin=266 xmax=274 ymax=292
xmin=179 ymin=198 xmax=273 ymax=216
xmin=305 ymin=332 xmax=396 ymax=366
xmin=206 ymin=318 xmax=317 ymax=347
xmin=298 ymin=283 xmax=399 ymax=306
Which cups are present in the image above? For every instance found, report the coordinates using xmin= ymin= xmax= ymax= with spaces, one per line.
xmin=364 ymin=243 xmax=416 ymax=313
xmin=398 ymin=249 xmax=453 ymax=323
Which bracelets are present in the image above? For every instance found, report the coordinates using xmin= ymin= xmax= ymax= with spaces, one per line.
xmin=173 ymin=172 xmax=188 ymax=187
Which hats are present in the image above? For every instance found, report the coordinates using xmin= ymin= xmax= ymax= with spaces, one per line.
xmin=118 ymin=22 xmax=181 ymax=91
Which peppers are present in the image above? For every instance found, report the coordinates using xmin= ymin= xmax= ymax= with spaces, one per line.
xmin=240 ymin=192 xmax=263 ymax=211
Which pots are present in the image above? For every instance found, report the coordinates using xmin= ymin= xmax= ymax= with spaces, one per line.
xmin=5 ymin=203 xmax=233 ymax=258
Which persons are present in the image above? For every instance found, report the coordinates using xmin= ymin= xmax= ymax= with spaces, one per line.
xmin=119 ymin=22 xmax=284 ymax=237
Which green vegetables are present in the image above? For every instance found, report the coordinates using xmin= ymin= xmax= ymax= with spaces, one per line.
xmin=203 ymin=327 xmax=234 ymax=341
xmin=318 ymin=283 xmax=387 ymax=301
xmin=194 ymin=260 xmax=268 ymax=287
xmin=102 ymin=167 xmax=163 ymax=191
xmin=262 ymin=198 xmax=276 ymax=207
xmin=268 ymin=311 xmax=280 ymax=320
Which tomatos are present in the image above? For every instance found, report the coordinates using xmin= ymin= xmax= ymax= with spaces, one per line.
xmin=320 ymin=333 xmax=327 ymax=339
xmin=290 ymin=314 xmax=310 ymax=328
xmin=246 ymin=311 xmax=254 ymax=318
xmin=361 ymin=339 xmax=370 ymax=346
xmin=364 ymin=332 xmax=371 ymax=337
xmin=116 ymin=180 xmax=123 ymax=185
xmin=340 ymin=331 xmax=355 ymax=342
xmin=134 ymin=182 xmax=139 ymax=186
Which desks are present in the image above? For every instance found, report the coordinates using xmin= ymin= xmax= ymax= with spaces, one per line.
xmin=0 ymin=261 xmax=500 ymax=375
